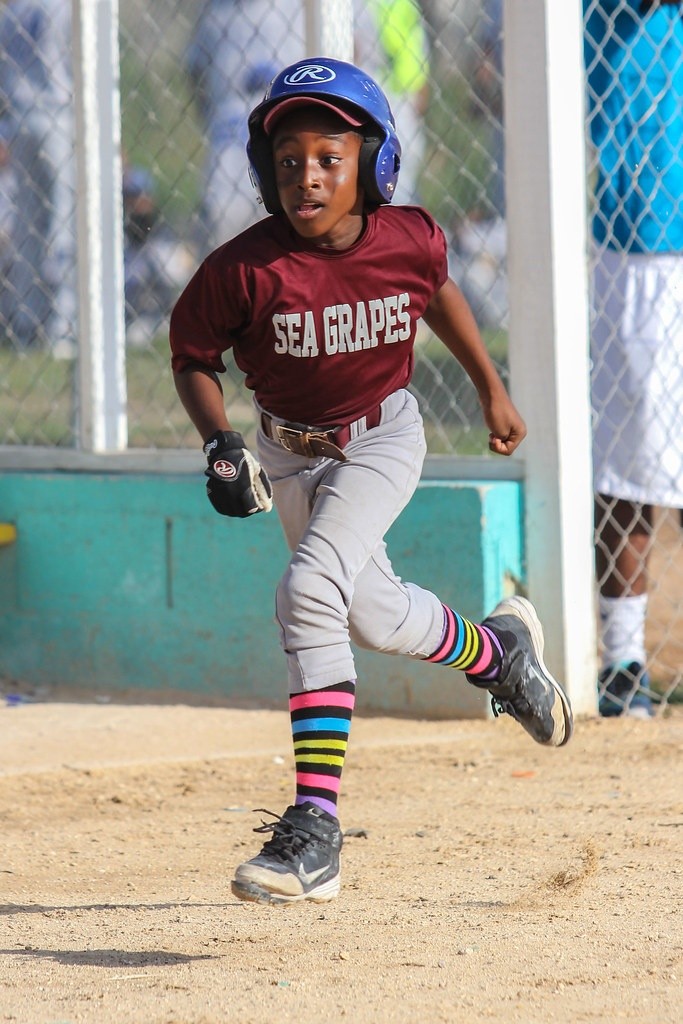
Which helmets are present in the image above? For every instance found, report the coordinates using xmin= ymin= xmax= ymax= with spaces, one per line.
xmin=245 ymin=57 xmax=401 ymax=214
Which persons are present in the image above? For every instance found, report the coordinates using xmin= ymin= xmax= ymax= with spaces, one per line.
xmin=0 ymin=0 xmax=504 ymax=360
xmin=170 ymin=57 xmax=572 ymax=906
xmin=581 ymin=0 xmax=683 ymax=719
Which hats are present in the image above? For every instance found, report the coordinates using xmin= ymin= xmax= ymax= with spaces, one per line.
xmin=263 ymin=94 xmax=369 ymax=135
xmin=122 ymin=167 xmax=153 ymax=196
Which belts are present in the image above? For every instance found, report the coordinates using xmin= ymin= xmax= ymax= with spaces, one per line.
xmin=261 ymin=404 xmax=381 ymax=461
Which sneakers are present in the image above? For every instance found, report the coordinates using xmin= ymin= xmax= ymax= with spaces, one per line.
xmin=597 ymin=660 xmax=654 ymax=719
xmin=231 ymin=800 xmax=344 ymax=906
xmin=465 ymin=596 xmax=573 ymax=747
